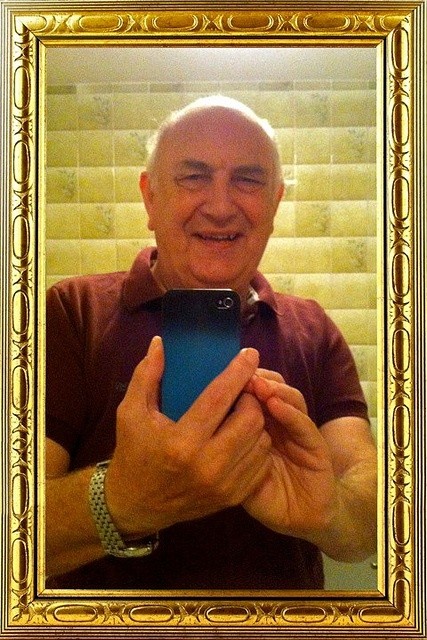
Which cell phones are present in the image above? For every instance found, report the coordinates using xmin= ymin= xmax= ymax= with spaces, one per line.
xmin=157 ymin=287 xmax=241 ymax=435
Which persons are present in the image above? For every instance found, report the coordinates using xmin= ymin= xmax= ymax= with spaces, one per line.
xmin=46 ymin=93 xmax=377 ymax=589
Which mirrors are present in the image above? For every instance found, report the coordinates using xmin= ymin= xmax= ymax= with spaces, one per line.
xmin=1 ymin=1 xmax=426 ymax=636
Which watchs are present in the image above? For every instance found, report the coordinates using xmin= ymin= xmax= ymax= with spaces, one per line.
xmin=88 ymin=460 xmax=161 ymax=560
